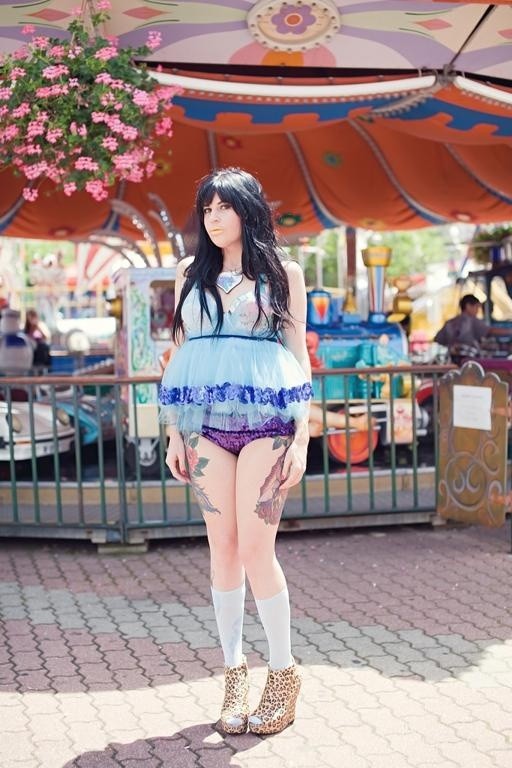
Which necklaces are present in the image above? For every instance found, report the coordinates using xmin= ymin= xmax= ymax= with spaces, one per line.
xmin=215 ymin=265 xmax=244 ymax=294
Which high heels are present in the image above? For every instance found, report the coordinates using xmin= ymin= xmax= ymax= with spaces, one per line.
xmin=221 ymin=653 xmax=300 ymax=734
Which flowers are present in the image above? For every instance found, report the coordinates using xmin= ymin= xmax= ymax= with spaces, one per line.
xmin=0 ymin=1 xmax=186 ymax=205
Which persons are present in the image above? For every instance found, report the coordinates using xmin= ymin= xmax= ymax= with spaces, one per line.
xmin=155 ymin=165 xmax=313 ymax=739
xmin=433 ymin=294 xmax=512 ymax=360
xmin=26 ymin=310 xmax=51 ymax=398
xmin=308 ymin=399 xmax=379 ymax=438
